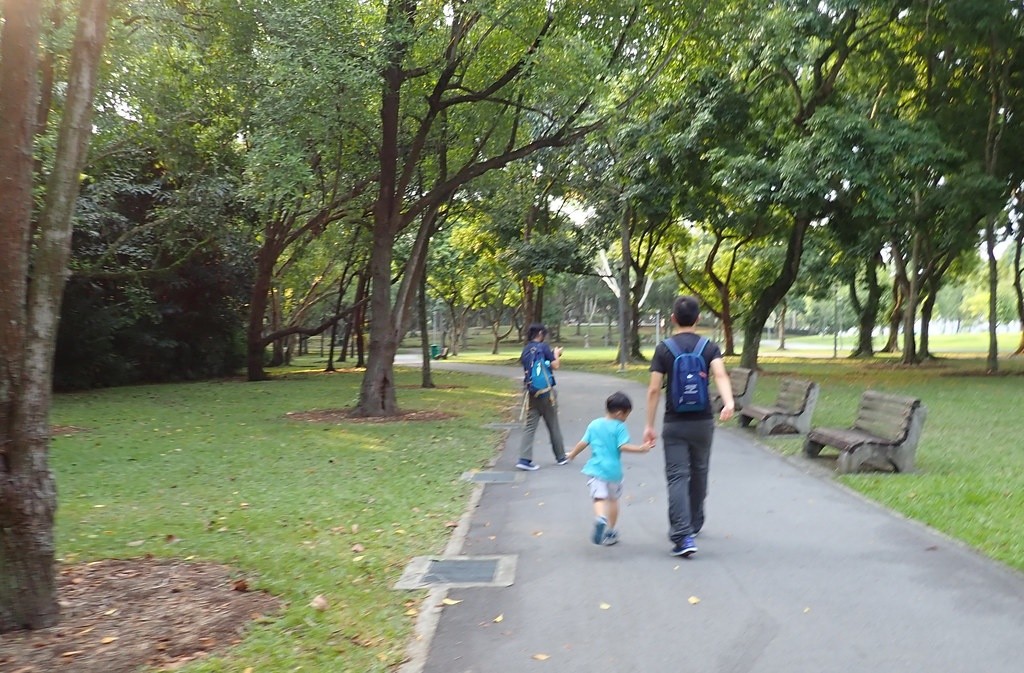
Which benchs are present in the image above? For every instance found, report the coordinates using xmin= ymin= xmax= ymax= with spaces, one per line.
xmin=706 ymin=366 xmax=758 ymax=412
xmin=434 ymin=347 xmax=449 ymax=360
xmin=740 ymin=373 xmax=820 ymax=438
xmin=802 ymin=390 xmax=927 ymax=475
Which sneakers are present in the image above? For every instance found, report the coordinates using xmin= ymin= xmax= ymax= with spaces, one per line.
xmin=558 ymin=452 xmax=570 ymax=464
xmin=670 ymin=537 xmax=697 ymax=557
xmin=516 ymin=459 xmax=540 ymax=470
xmin=690 ymin=529 xmax=700 ymax=537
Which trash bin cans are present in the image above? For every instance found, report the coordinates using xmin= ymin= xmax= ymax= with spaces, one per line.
xmin=431 ymin=344 xmax=440 ymax=359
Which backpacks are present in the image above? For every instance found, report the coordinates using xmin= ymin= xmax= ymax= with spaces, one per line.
xmin=663 ymin=336 xmax=709 ymax=414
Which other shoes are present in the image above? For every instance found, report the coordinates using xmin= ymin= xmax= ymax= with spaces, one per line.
xmin=594 ymin=518 xmax=606 ymax=545
xmin=602 ymin=530 xmax=619 ymax=545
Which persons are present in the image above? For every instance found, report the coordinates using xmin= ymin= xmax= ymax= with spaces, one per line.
xmin=642 ymin=295 xmax=735 ymax=560
xmin=565 ymin=390 xmax=657 ymax=546
xmin=515 ymin=322 xmax=572 ymax=470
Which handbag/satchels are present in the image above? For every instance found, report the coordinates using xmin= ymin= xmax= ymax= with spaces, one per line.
xmin=527 ymin=343 xmax=553 ymax=398
